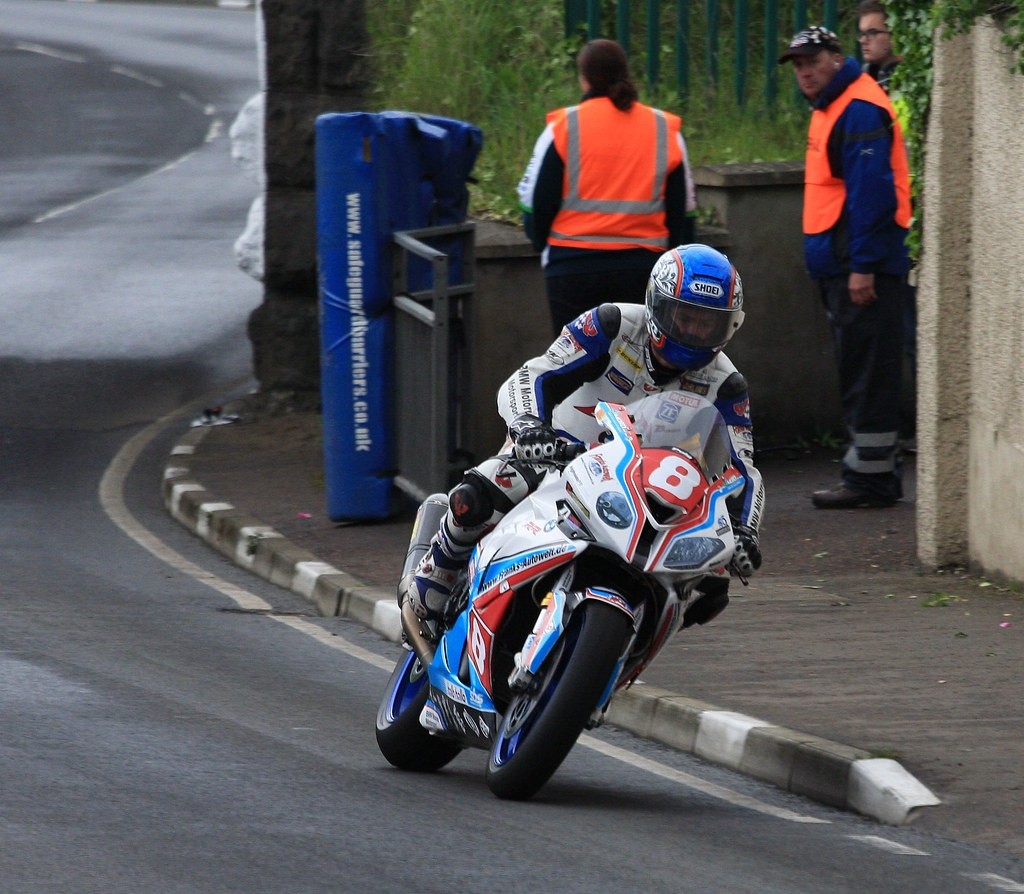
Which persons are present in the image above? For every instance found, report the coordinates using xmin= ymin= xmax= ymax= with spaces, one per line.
xmin=775 ymin=0 xmax=912 ymax=508
xmin=409 ymin=243 xmax=764 ymax=619
xmin=518 ymin=41 xmax=697 ymax=342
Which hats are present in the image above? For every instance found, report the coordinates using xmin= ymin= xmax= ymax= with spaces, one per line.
xmin=776 ymin=25 xmax=842 ymax=65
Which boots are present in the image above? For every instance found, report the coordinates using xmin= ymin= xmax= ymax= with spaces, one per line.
xmin=408 ymin=504 xmax=478 ymax=621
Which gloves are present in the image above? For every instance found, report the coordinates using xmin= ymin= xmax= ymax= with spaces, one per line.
xmin=725 ymin=523 xmax=762 ymax=577
xmin=510 ymin=416 xmax=558 ymax=475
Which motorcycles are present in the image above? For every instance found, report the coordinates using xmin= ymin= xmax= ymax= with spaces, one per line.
xmin=376 ymin=390 xmax=749 ymax=801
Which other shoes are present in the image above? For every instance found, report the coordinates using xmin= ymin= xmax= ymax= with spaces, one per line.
xmin=810 ymin=479 xmax=900 ymax=509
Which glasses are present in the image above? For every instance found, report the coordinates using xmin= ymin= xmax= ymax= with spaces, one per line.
xmin=854 ymin=28 xmax=893 ymax=42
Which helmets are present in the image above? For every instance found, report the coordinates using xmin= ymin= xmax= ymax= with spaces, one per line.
xmin=644 ymin=243 xmax=745 ymax=370
